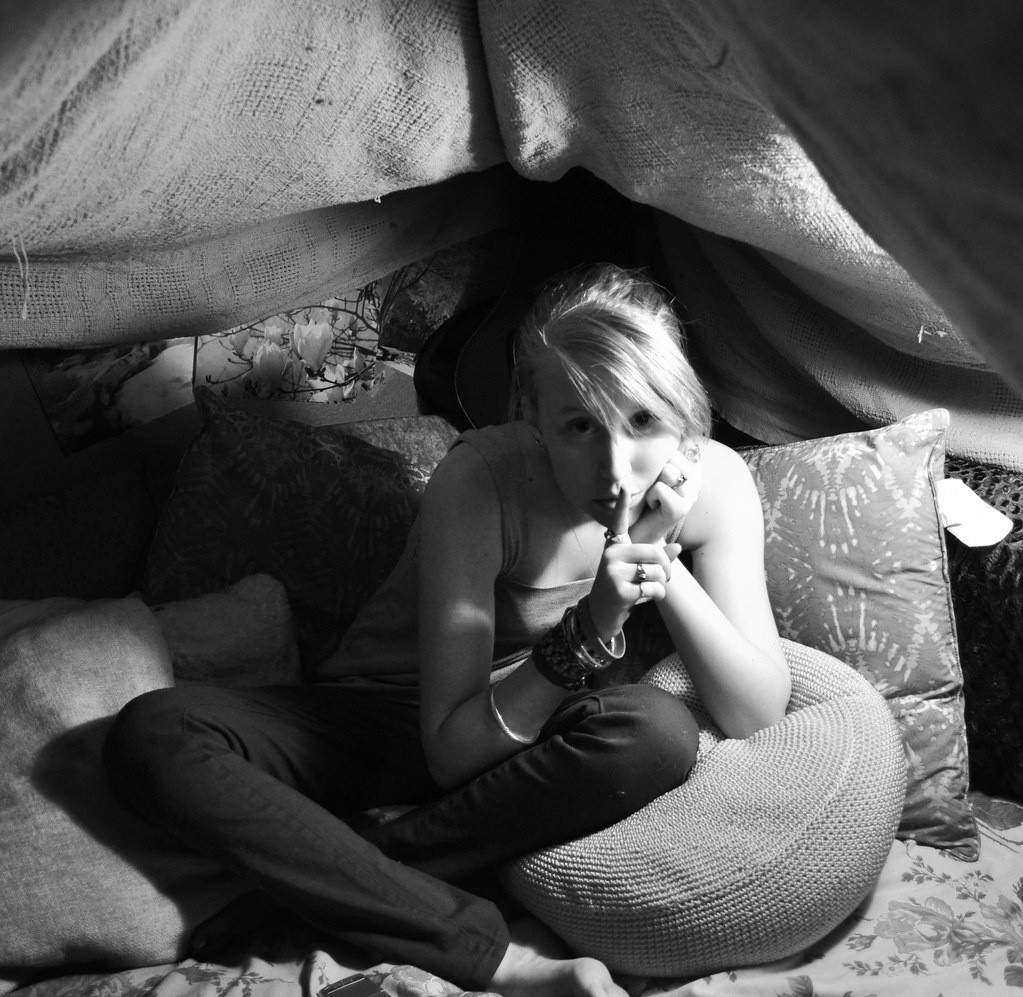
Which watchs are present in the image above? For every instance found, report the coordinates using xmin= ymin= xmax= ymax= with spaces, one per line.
xmin=540 ymin=628 xmax=603 ymax=692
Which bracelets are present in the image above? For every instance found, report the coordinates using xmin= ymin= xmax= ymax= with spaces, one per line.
xmin=488 ymin=679 xmax=541 ymax=745
xmin=530 ymin=595 xmax=626 ymax=694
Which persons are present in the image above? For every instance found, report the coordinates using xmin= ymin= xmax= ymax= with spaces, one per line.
xmin=101 ymin=266 xmax=793 ymax=996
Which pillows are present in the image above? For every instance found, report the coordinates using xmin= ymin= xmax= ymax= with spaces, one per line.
xmin=148 ymin=568 xmax=307 ymax=690
xmin=0 ymin=591 xmax=242 ymax=966
xmin=730 ymin=408 xmax=988 ymax=864
xmin=135 ymin=385 xmax=435 ymax=686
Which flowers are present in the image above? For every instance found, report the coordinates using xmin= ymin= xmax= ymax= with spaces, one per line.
xmin=191 ymin=280 xmax=418 ymax=401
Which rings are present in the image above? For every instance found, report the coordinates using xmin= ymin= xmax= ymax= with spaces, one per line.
xmin=639 ymin=584 xmax=646 ymax=597
xmin=604 ymin=531 xmax=630 ymax=541
xmin=635 ymin=562 xmax=647 ymax=584
xmin=670 ymin=474 xmax=687 ymax=490
